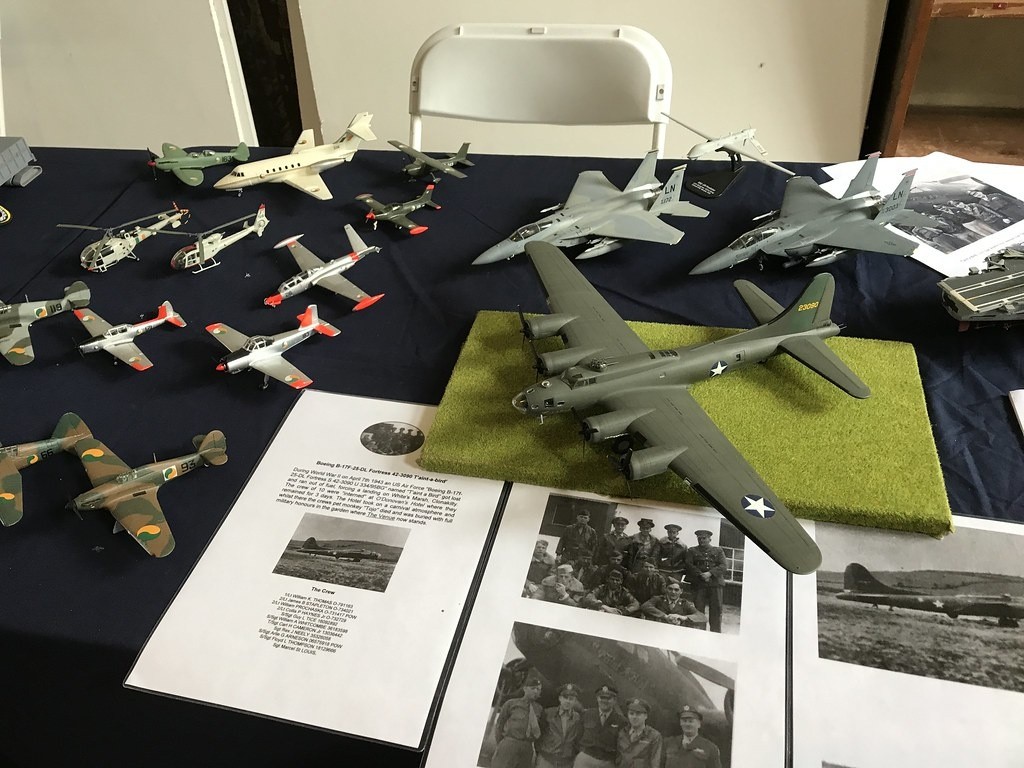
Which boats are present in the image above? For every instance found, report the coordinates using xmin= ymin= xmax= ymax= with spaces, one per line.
xmin=937 ymin=248 xmax=1023 ymax=333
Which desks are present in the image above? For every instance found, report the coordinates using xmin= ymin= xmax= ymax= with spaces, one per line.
xmin=0 ymin=145 xmax=1024 ymax=768
xmin=0 ymin=147 xmax=159 ymax=304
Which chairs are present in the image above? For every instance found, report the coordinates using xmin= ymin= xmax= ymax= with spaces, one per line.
xmin=409 ymin=21 xmax=673 ymax=159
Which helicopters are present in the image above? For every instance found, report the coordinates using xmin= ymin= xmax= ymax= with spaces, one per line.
xmin=56 ymin=201 xmax=190 ymax=274
xmin=137 ymin=204 xmax=270 ymax=274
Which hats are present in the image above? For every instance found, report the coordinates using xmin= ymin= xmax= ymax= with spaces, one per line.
xmin=526 ymin=677 xmax=541 ymax=686
xmin=391 ymin=427 xmax=397 ymax=429
xmin=626 ymin=696 xmax=651 ymax=713
xmin=408 ymin=429 xmax=413 ymax=431
xmin=557 ymin=564 xmax=573 ymax=573
xmin=609 ymin=570 xmax=623 ymax=579
xmin=644 ymin=557 xmax=656 ymax=566
xmin=611 ymin=517 xmax=629 ymax=525
xmin=581 ymin=549 xmax=594 ymax=557
xmin=595 ymin=683 xmax=618 ymax=698
xmin=557 ymin=683 xmax=580 ymax=696
xmin=400 ymin=428 xmax=405 ymax=430
xmin=417 ymin=431 xmax=421 ymax=433
xmin=380 ymin=425 xmax=384 ymax=427
xmin=695 ymin=530 xmax=713 ymax=536
xmin=577 ymin=510 xmax=591 ymax=516
xmin=677 ymin=704 xmax=704 ymax=721
xmin=611 ymin=551 xmax=621 ymax=556
xmin=637 ymin=518 xmax=655 ymax=527
xmin=666 ymin=576 xmax=679 ymax=586
xmin=664 ymin=524 xmax=682 ymax=531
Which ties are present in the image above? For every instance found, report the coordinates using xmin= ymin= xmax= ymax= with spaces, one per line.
xmin=631 ymin=730 xmax=638 ymax=742
xmin=526 ymin=701 xmax=541 ymax=739
xmin=669 ymin=602 xmax=675 ymax=611
xmin=601 ymin=713 xmax=606 ymax=725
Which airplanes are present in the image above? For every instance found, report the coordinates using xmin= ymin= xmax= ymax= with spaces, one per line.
xmin=660 ymin=111 xmax=797 ymax=177
xmin=63 ymin=285 xmax=188 ymax=371
xmin=0 ymin=280 xmax=91 ymax=366
xmin=508 ymin=240 xmax=874 ymax=577
xmin=836 ymin=561 xmax=1024 ymax=628
xmin=262 ymin=223 xmax=387 ymax=313
xmin=50 ymin=412 xmax=230 ymax=558
xmin=489 ymin=622 xmax=736 ymax=762
xmin=295 ymin=536 xmax=383 ymax=562
xmin=688 ymin=151 xmax=939 ymax=277
xmin=469 ymin=149 xmax=710 ymax=266
xmin=354 ymin=184 xmax=442 ymax=235
xmin=211 ymin=110 xmax=378 ymax=201
xmin=0 ymin=433 xmax=84 ymax=527
xmin=388 ymin=139 xmax=477 ymax=184
xmin=203 ymin=303 xmax=342 ymax=390
xmin=145 ymin=141 xmax=250 ymax=187
xmin=885 ymin=173 xmax=987 ymax=223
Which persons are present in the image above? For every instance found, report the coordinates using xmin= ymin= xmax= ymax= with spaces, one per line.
xmin=651 ymin=524 xmax=687 ymax=580
xmin=593 ymin=518 xmax=633 ymax=579
xmin=630 ymin=557 xmax=667 ymax=605
xmin=911 ymin=189 xmax=1024 ymax=254
xmin=556 ymin=509 xmax=598 ymax=578
xmin=660 ymin=704 xmax=723 ymax=768
xmin=640 ymin=575 xmax=709 ymax=631
xmin=522 ymin=540 xmax=554 ymax=603
xmin=630 ymin=519 xmax=661 ymax=570
xmin=589 ymin=550 xmax=632 ymax=590
xmin=684 ymin=530 xmax=727 ymax=633
xmin=531 ymin=563 xmax=585 ymax=608
xmin=490 ymin=666 xmax=545 ymax=768
xmin=535 ymin=683 xmax=583 ymax=768
xmin=585 ymin=569 xmax=640 ymax=618
xmin=615 ymin=697 xmax=664 ymax=768
xmin=573 ymin=684 xmax=631 ymax=768
